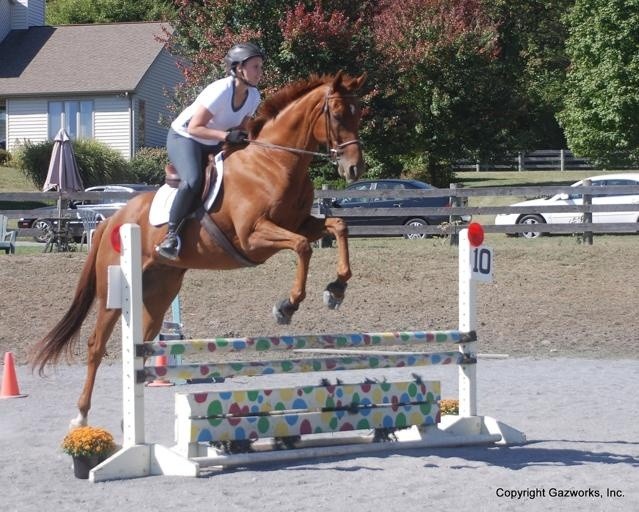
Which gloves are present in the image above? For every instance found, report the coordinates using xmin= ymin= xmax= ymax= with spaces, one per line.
xmin=226 ymin=130 xmax=248 ymax=146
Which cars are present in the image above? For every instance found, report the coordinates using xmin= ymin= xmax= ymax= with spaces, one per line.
xmin=17 ymin=184 xmax=158 ymax=242
xmin=312 ymin=178 xmax=472 ymax=240
xmin=494 ymin=172 xmax=638 ymax=239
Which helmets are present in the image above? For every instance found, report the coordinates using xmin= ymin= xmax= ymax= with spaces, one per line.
xmin=223 ymin=42 xmax=265 ymax=79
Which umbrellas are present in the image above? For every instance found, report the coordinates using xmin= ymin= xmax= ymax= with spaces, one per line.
xmin=42 ymin=130 xmax=84 ymax=250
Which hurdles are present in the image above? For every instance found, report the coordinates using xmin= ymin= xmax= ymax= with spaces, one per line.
xmin=89 ymin=224 xmax=527 ymax=482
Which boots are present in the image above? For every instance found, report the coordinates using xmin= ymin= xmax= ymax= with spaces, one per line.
xmin=161 ymin=222 xmax=178 ymax=250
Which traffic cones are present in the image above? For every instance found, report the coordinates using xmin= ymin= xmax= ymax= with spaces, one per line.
xmin=0 ymin=351 xmax=20 ymax=396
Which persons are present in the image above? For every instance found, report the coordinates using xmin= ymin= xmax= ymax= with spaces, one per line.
xmin=159 ymin=42 xmax=263 ymax=261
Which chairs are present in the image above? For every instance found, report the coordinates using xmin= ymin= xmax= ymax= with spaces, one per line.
xmin=0 ymin=215 xmax=16 ymax=256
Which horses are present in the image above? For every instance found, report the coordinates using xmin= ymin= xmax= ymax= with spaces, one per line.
xmin=29 ymin=69 xmax=367 ymax=432
